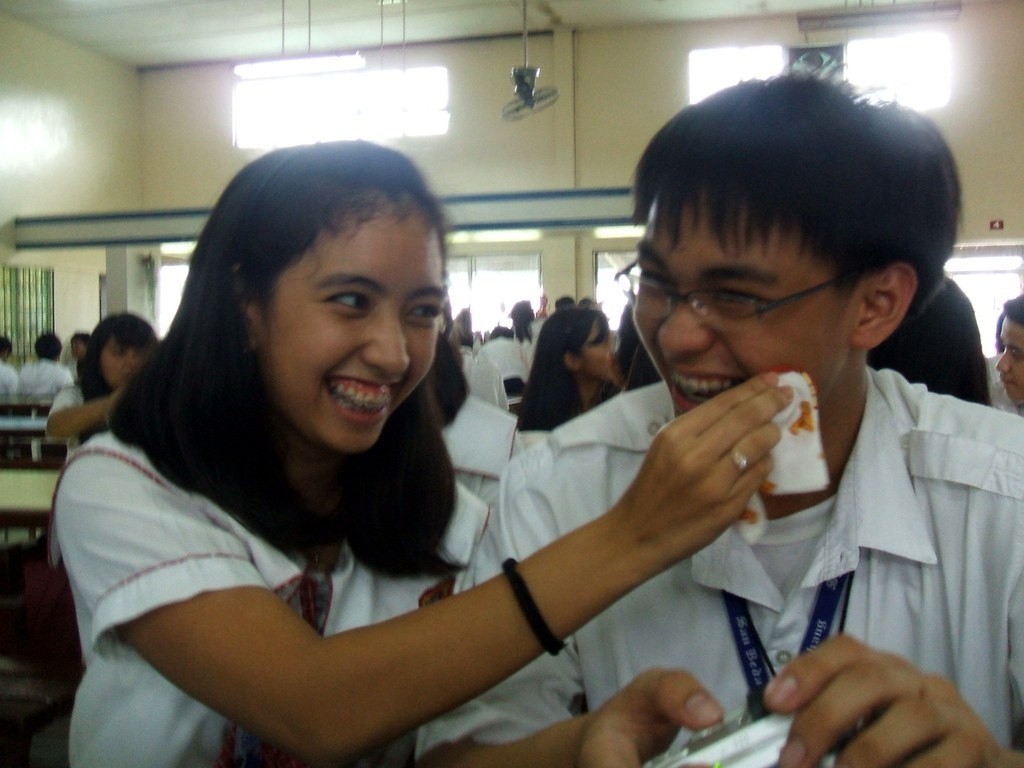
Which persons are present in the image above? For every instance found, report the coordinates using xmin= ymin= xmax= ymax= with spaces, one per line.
xmin=53 ymin=140 xmax=794 ymax=768
xmin=0 ymin=296 xmax=1024 ymax=492
xmin=417 ymin=69 xmax=1024 ymax=767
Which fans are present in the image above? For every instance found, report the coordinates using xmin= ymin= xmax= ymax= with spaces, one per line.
xmin=501 ymin=1 xmax=559 ymax=121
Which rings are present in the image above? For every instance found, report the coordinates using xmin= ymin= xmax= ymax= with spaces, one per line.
xmin=731 ymin=450 xmax=747 ymax=473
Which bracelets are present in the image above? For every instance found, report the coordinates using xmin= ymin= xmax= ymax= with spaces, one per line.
xmin=503 ymin=559 xmax=566 ymax=655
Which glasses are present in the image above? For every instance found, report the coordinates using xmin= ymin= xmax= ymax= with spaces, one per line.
xmin=613 ymin=260 xmax=864 ymax=329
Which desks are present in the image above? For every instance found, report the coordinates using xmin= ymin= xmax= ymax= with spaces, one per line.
xmin=0 ymin=416 xmax=60 ymax=648
